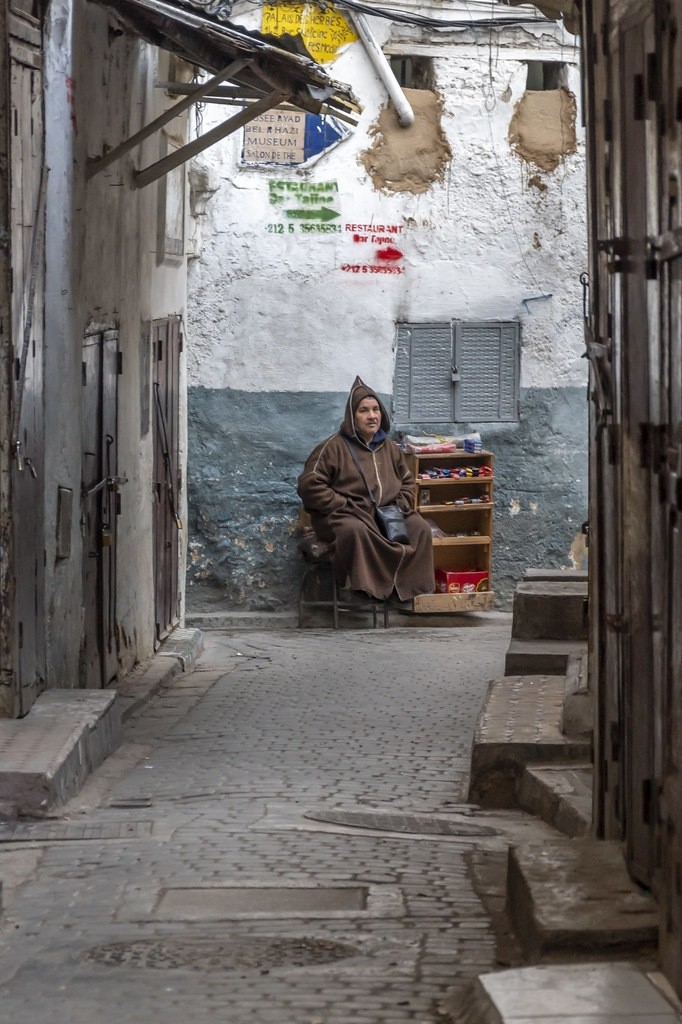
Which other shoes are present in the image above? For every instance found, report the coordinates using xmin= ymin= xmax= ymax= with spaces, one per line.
xmin=337 ymin=590 xmax=353 ymax=612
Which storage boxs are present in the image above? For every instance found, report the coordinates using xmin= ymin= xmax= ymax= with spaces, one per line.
xmin=434 ymin=566 xmax=489 ymax=593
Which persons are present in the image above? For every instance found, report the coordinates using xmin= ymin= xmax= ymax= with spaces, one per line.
xmin=299 ymin=376 xmax=434 ymax=607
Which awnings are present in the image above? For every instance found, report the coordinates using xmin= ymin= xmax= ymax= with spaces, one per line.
xmin=92 ymin=0 xmax=364 ymax=190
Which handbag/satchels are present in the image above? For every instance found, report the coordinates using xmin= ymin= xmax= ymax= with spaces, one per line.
xmin=375 ymin=507 xmax=410 ymax=545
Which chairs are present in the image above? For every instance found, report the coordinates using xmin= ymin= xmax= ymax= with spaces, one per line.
xmin=296 ymin=503 xmax=390 ymax=631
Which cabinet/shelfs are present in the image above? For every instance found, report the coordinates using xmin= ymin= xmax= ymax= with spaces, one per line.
xmin=401 ymin=449 xmax=493 ymax=612
